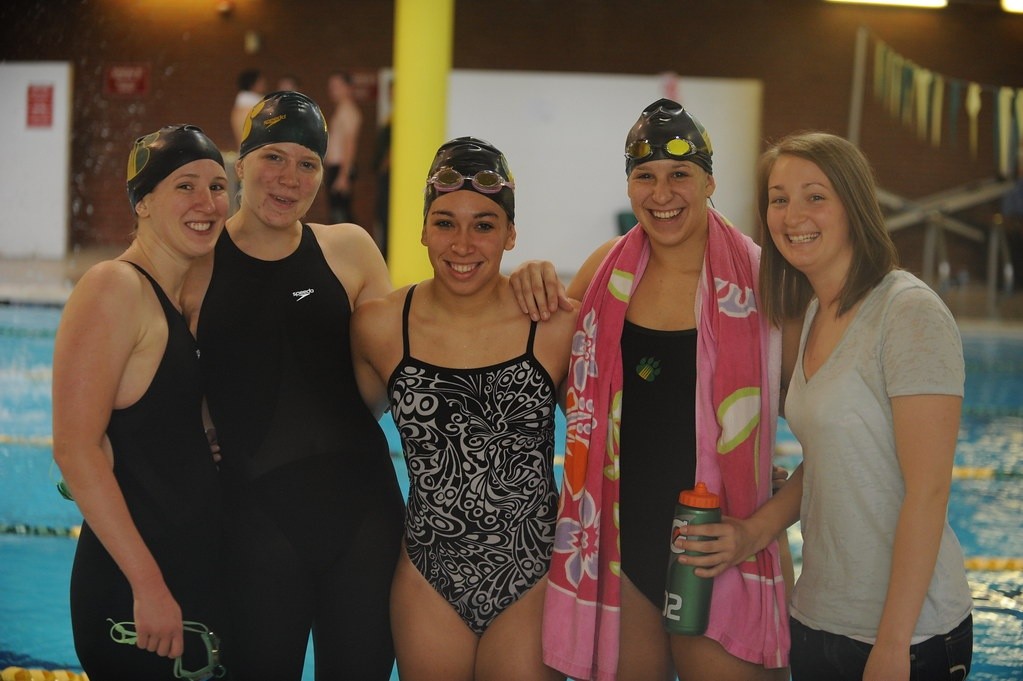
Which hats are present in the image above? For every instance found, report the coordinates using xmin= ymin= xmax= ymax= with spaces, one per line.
xmin=626 ymin=98 xmax=713 ymax=176
xmin=425 ymin=136 xmax=515 ymax=222
xmin=126 ymin=123 xmax=226 ymax=210
xmin=238 ymin=90 xmax=328 ymax=164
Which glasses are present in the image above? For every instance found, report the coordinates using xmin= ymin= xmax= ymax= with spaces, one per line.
xmin=427 ymin=169 xmax=503 ymax=194
xmin=625 ymin=137 xmax=697 ymax=162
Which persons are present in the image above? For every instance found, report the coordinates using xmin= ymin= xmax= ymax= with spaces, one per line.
xmin=212 ymin=136 xmax=788 ymax=681
xmin=374 ymin=81 xmax=394 ymax=204
xmin=508 ymin=98 xmax=804 ymax=681
xmin=231 ymin=69 xmax=266 ymax=145
xmin=755 ymin=133 xmax=974 ymax=681
xmin=321 ymin=70 xmax=365 ymax=226
xmin=179 ymin=91 xmax=406 ymax=681
xmin=52 ymin=125 xmax=230 ymax=681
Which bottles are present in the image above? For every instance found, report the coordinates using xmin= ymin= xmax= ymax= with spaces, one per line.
xmin=662 ymin=482 xmax=721 ymax=635
